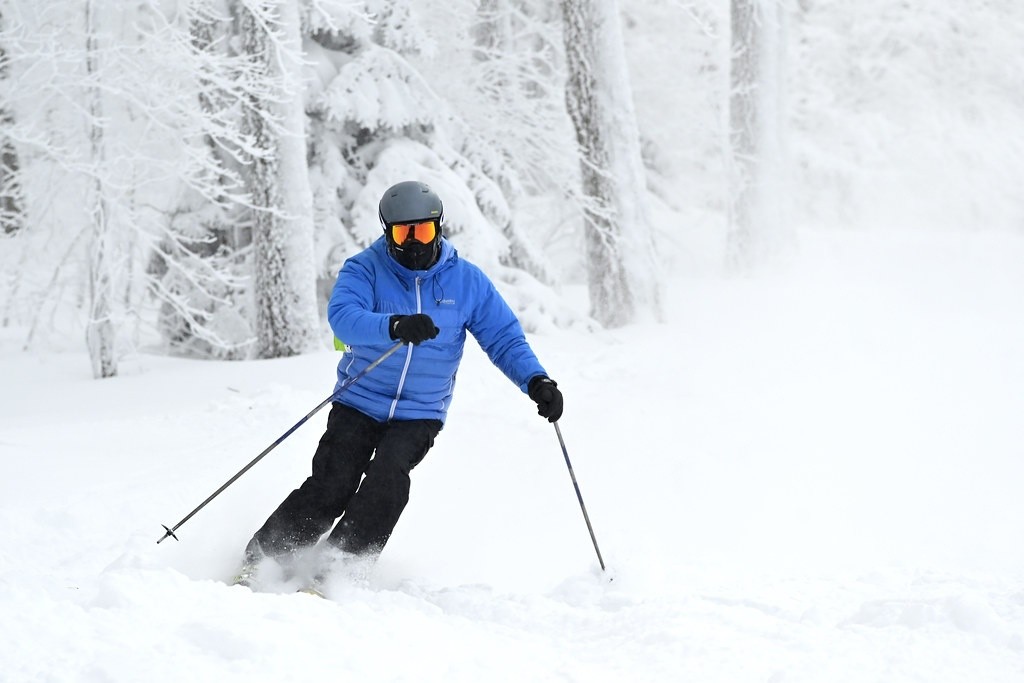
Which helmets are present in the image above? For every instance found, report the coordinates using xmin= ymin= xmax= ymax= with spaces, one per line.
xmin=378 ymin=181 xmax=444 ymax=271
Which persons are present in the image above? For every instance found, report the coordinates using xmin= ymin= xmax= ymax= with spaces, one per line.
xmin=234 ymin=180 xmax=564 ymax=601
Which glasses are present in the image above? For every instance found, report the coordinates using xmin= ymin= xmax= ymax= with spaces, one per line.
xmin=389 ymin=219 xmax=438 ymax=248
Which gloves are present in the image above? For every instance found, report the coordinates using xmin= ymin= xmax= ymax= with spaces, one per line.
xmin=528 ymin=375 xmax=563 ymax=423
xmin=389 ymin=314 xmax=440 ymax=346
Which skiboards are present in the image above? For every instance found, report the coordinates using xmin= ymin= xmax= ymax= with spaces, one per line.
xmin=233 ymin=578 xmax=328 ymax=599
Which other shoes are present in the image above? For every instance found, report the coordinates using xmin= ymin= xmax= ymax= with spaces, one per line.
xmin=308 ymin=575 xmax=326 ymax=598
xmin=241 ymin=538 xmax=267 ymax=580
